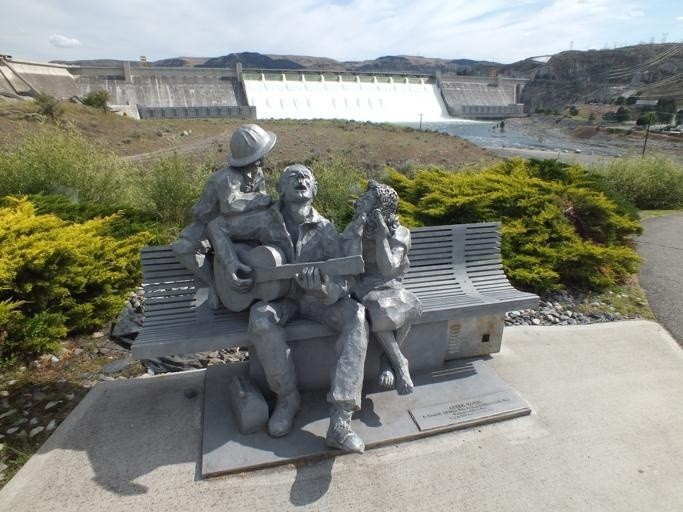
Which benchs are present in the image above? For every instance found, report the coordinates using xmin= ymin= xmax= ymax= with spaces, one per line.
xmin=130 ymin=214 xmax=541 ymax=394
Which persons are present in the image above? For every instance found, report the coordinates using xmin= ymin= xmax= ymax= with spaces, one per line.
xmin=173 ymin=123 xmax=278 ymax=311
xmin=205 ymin=161 xmax=371 ymax=456
xmin=339 ymin=179 xmax=423 ymax=395
